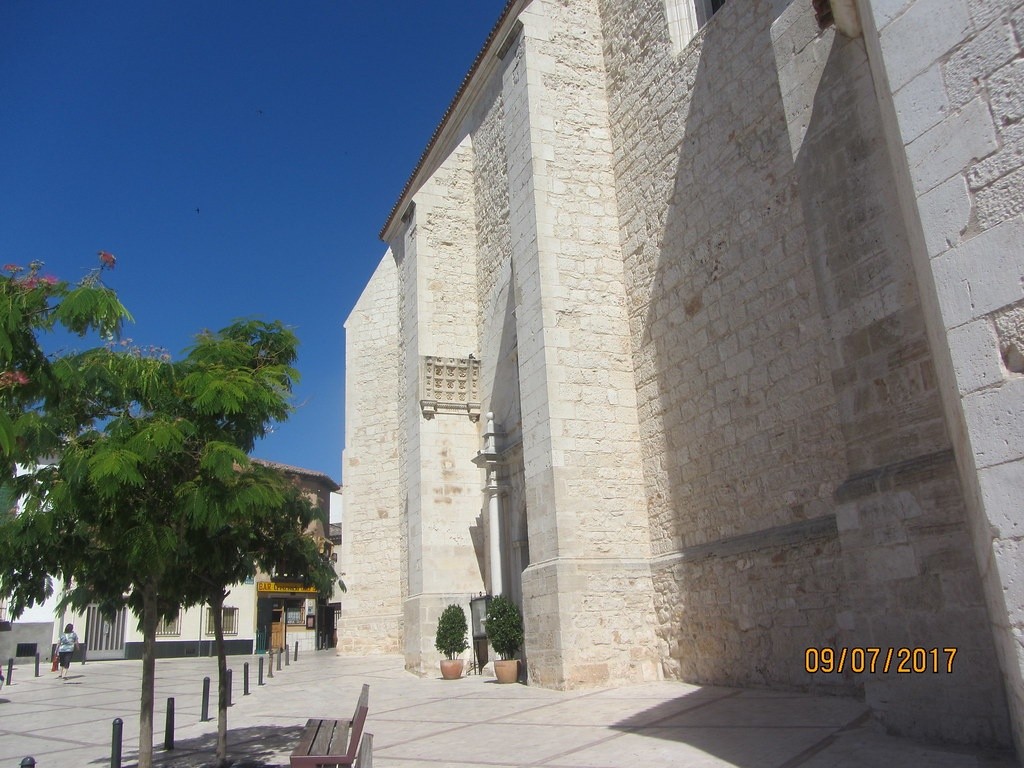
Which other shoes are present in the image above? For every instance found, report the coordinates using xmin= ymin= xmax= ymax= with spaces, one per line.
xmin=63 ymin=676 xmax=69 ymax=680
xmin=55 ymin=673 xmax=62 ymax=679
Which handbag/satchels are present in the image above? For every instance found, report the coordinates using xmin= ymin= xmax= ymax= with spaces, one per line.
xmin=52 ymin=653 xmax=59 ymax=672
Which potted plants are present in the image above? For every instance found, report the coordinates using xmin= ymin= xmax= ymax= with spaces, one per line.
xmin=434 ymin=604 xmax=471 ymax=680
xmin=484 ymin=594 xmax=524 ymax=685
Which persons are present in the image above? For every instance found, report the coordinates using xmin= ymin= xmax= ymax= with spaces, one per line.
xmin=55 ymin=624 xmax=80 ymax=680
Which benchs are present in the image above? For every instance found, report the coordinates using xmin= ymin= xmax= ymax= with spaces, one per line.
xmin=289 ymin=684 xmax=370 ymax=768
